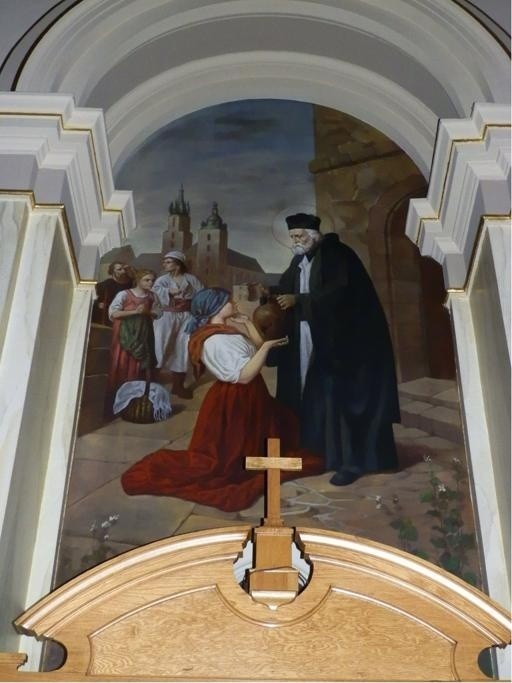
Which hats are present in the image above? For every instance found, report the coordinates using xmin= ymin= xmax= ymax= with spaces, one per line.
xmin=286 ymin=213 xmax=321 ymax=232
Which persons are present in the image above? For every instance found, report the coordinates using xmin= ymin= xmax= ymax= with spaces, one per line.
xmin=120 ymin=286 xmax=326 ymax=512
xmin=94 ymin=260 xmax=137 ymax=308
xmin=260 ymin=211 xmax=404 ymax=486
xmin=104 ymin=269 xmax=163 ymax=419
xmin=151 ymin=251 xmax=206 ymax=399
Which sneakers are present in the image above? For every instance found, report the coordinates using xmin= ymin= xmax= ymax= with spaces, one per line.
xmin=331 ymin=469 xmax=360 ymax=486
